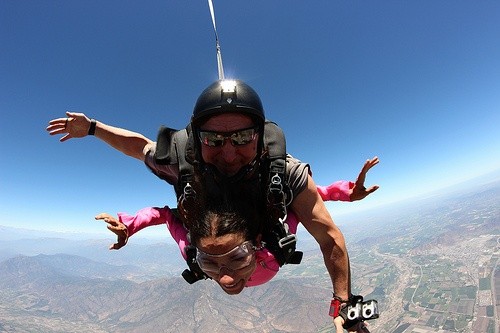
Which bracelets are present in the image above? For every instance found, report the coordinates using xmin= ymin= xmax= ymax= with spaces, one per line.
xmin=88 ymin=118 xmax=97 ymax=136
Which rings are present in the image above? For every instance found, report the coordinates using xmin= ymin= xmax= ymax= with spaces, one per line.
xmin=66 ymin=118 xmax=68 ymax=123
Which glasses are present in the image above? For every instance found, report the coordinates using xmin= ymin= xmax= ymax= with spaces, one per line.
xmin=197 ymin=123 xmax=257 ymax=147
xmin=195 ymin=240 xmax=253 ymax=274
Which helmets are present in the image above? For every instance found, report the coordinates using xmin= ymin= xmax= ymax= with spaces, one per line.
xmin=190 ymin=79 xmax=265 ymax=173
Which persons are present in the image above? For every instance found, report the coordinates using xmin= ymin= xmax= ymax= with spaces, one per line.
xmin=45 ymin=78 xmax=371 ymax=333
xmin=94 ymin=156 xmax=381 ymax=295
